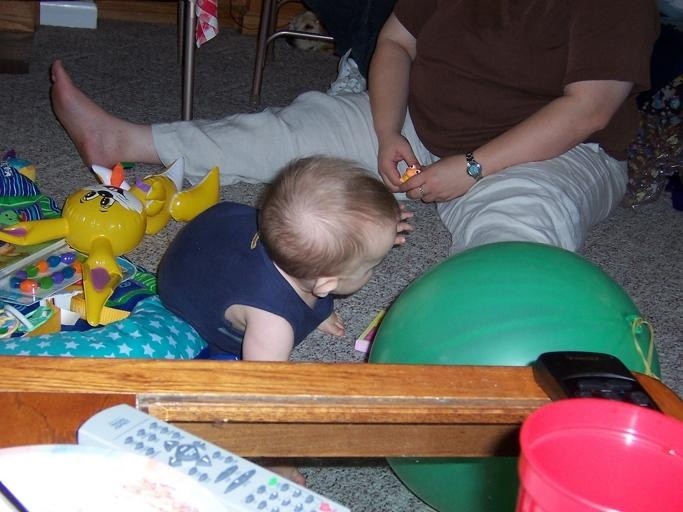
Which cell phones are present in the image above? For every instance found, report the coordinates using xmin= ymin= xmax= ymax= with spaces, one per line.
xmin=540 ymin=350 xmax=664 ymax=414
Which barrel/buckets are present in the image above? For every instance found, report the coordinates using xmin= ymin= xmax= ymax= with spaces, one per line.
xmin=513 ymin=399 xmax=682 ymax=512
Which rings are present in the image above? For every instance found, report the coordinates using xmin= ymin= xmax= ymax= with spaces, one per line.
xmin=418 ymin=186 xmax=424 ymax=195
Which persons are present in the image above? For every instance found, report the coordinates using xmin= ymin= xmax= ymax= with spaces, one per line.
xmin=155 ymin=156 xmax=414 ymax=362
xmin=45 ymin=0 xmax=662 ymax=257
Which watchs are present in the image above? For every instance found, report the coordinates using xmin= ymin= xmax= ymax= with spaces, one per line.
xmin=464 ymin=149 xmax=484 ymax=181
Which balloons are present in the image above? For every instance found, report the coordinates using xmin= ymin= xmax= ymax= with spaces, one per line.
xmin=365 ymin=237 xmax=662 ymax=512
